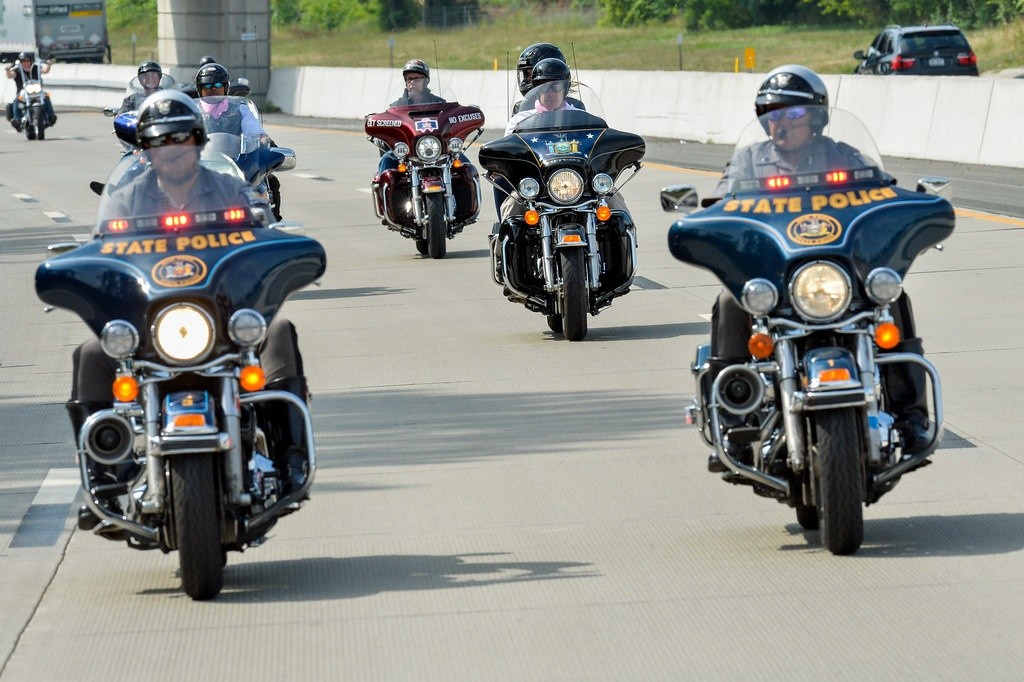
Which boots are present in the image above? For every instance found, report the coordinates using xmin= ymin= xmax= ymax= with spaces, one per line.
xmin=877 ymin=337 xmax=931 ymax=453
xmin=65 ymin=399 xmax=124 ymax=530
xmin=265 ymin=376 xmax=311 ymax=502
xmin=708 ymin=357 xmax=755 ymax=472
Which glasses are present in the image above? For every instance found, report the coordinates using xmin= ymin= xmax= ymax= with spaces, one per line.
xmin=143 ymin=131 xmax=192 ymax=147
xmin=766 ymin=104 xmax=807 ymax=121
xmin=406 ymin=76 xmax=423 ymax=83
xmin=202 ymin=82 xmax=224 ymax=89
xmin=538 ymin=81 xmax=562 ymax=92
xmin=23 ymin=60 xmax=29 ymax=62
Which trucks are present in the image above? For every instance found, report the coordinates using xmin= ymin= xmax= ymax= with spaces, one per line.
xmin=0 ymin=0 xmax=110 ymax=65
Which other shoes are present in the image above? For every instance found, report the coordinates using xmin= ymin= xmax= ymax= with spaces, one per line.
xmin=49 ymin=115 xmax=57 ymax=127
xmin=11 ymin=119 xmax=21 ymax=132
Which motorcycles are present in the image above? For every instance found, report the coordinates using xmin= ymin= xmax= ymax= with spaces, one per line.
xmin=3 ymin=57 xmax=59 ymax=139
xmin=361 ymin=76 xmax=487 ymax=260
xmin=102 ymin=70 xmax=189 ymax=162
xmin=476 ymin=76 xmax=650 ymax=343
xmin=31 ymin=143 xmax=331 ymax=604
xmin=660 ymin=103 xmax=959 ymax=556
xmin=88 ymin=92 xmax=298 ymax=227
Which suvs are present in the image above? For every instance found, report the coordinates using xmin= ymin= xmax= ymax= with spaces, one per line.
xmin=852 ymin=21 xmax=981 ymax=77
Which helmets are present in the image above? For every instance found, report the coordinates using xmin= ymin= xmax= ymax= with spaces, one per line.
xmin=531 ymin=58 xmax=571 ymax=98
xmin=755 ymin=65 xmax=828 ymax=140
xmin=516 ymin=43 xmax=571 ymax=100
xmin=196 ymin=63 xmax=231 ymax=104
xmin=200 ymin=56 xmax=217 ymax=67
xmin=403 ymin=59 xmax=430 ymax=82
xmin=136 ymin=89 xmax=207 ymax=150
xmin=138 ymin=61 xmax=162 ymax=89
xmin=18 ymin=51 xmax=33 ymax=65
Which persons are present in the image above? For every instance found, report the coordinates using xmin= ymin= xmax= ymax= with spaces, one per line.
xmin=510 ymin=43 xmax=586 ymax=115
xmin=5 ymin=52 xmax=56 ymax=132
xmin=376 ymin=59 xmax=470 ymax=173
xmin=65 ymin=90 xmax=308 ymax=539
xmin=491 ymin=57 xmax=637 ymax=251
xmin=709 ymin=64 xmax=939 ymax=472
xmin=195 ymin=64 xmax=282 ymax=221
xmin=116 ymin=61 xmax=161 ymax=114
